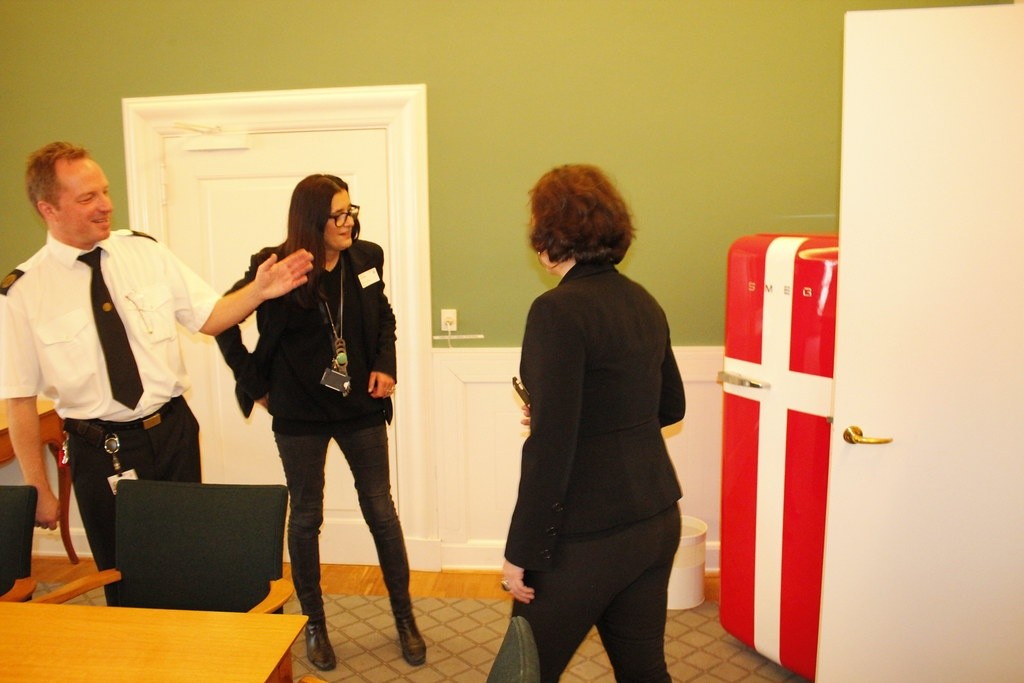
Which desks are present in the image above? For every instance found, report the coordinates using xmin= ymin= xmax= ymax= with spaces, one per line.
xmin=0 ymin=397 xmax=79 ymax=564
xmin=0 ymin=601 xmax=308 ymax=683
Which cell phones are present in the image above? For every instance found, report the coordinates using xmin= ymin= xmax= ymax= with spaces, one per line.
xmin=512 ymin=377 xmax=531 ymax=409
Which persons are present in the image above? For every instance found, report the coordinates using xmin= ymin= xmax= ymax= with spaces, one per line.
xmin=0 ymin=141 xmax=315 ymax=606
xmin=495 ymin=161 xmax=686 ymax=683
xmin=212 ymin=171 xmax=428 ymax=671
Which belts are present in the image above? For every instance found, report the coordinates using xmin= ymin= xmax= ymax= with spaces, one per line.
xmin=92 ymin=400 xmax=176 ymax=432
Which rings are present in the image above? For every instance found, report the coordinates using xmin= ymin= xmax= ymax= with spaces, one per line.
xmin=500 ymin=580 xmax=512 ymax=592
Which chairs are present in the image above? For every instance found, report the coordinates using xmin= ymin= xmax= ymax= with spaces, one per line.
xmin=0 ymin=485 xmax=36 ymax=602
xmin=28 ymin=479 xmax=294 ymax=614
xmin=298 ymin=616 xmax=540 ymax=683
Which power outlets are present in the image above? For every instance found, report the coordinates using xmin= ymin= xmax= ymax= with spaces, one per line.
xmin=441 ymin=309 xmax=457 ymax=331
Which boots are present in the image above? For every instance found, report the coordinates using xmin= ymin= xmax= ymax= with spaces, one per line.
xmin=392 ymin=604 xmax=427 ymax=666
xmin=304 ymin=607 xmax=337 ymax=670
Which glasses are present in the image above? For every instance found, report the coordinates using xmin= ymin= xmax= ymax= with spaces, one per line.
xmin=329 ymin=204 xmax=360 ymax=227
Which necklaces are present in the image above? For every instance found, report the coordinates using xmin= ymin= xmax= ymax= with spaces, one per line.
xmin=325 ymin=270 xmax=349 ymax=375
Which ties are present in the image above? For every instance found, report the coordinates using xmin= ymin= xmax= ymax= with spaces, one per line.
xmin=77 ymin=247 xmax=145 ymax=410
